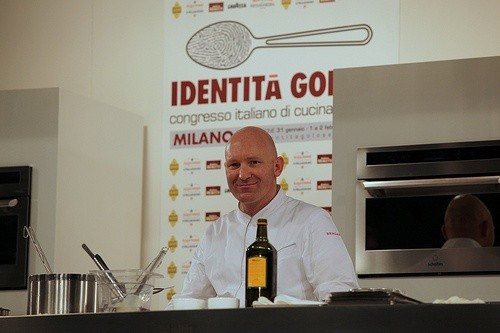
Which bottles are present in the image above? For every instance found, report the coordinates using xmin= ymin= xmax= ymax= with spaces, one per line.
xmin=245 ymin=219 xmax=277 ymax=308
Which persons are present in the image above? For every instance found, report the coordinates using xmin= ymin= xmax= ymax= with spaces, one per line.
xmin=397 ymin=194 xmax=495 ymax=270
xmin=178 ymin=125 xmax=361 ymax=306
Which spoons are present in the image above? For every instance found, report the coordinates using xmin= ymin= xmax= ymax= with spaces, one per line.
xmin=24 ymin=225 xmax=53 ymax=274
xmin=82 ymin=243 xmax=169 ymax=302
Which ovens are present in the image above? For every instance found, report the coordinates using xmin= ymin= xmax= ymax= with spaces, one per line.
xmin=332 ymin=56 xmax=499 ymax=305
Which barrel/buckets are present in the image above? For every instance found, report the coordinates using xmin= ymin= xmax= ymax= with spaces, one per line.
xmin=27 ymin=273 xmax=99 ymax=316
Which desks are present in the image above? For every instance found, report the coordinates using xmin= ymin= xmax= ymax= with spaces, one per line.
xmin=1 ymin=299 xmax=499 ymax=333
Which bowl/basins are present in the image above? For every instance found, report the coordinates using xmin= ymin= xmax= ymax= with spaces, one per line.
xmin=208 ymin=297 xmax=240 ymax=309
xmin=173 ymin=298 xmax=206 ymax=310
